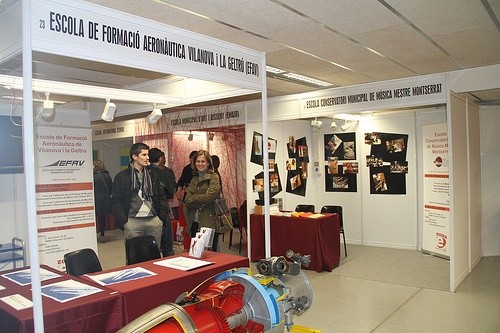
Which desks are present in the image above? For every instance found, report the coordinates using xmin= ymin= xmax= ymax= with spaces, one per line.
xmin=245 ymin=212 xmax=340 ymax=272
xmin=78 ymin=250 xmax=249 ymax=328
xmin=0 ymin=265 xmax=124 ymax=333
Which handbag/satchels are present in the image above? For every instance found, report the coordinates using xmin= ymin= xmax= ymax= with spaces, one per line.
xmin=208 ymin=173 xmax=233 ymax=233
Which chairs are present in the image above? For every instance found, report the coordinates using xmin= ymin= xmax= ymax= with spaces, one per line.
xmin=125 ymin=234 xmax=163 ymax=266
xmin=223 ymin=204 xmax=348 ymax=258
xmin=64 ymin=247 xmax=103 ymax=277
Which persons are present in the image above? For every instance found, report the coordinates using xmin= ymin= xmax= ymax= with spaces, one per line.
xmin=111 ymin=142 xmax=167 ymax=261
xmin=160 ymin=151 xmax=180 ymax=252
xmin=92 ymin=160 xmax=116 ymax=243
xmin=147 ymin=148 xmax=176 ymax=256
xmin=174 ymin=151 xmax=226 ymax=253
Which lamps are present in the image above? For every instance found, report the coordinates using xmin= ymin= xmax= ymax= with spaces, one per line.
xmin=146 ymin=102 xmax=163 ymax=125
xmin=329 ymin=118 xmax=337 ymax=131
xmin=40 ymin=92 xmax=55 ymax=118
xmin=208 ymin=130 xmax=214 ymax=141
xmin=310 ymin=117 xmax=322 ymax=129
xmin=221 ymin=131 xmax=228 ymax=141
xmin=340 ymin=119 xmax=351 ymax=131
xmin=188 ymin=130 xmax=193 ymax=141
xmin=101 ymin=98 xmax=117 ymax=123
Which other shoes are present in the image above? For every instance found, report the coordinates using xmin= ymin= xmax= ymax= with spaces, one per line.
xmin=100 ymin=234 xmax=105 ymax=242
